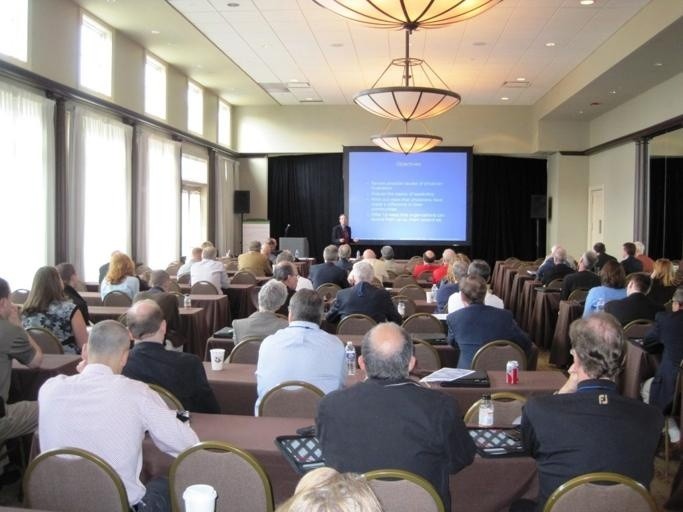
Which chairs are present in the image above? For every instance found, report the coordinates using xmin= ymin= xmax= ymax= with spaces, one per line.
xmin=19 ymin=447 xmax=130 ymax=512
xmin=11 ymin=288 xmax=30 ymax=303
xmin=640 ymin=359 xmax=683 ymax=477
xmin=171 ymin=439 xmax=275 ymax=512
xmin=142 ymin=271 xmax=153 ymax=287
xmin=276 ymin=313 xmax=288 ymax=320
xmin=417 ymin=270 xmax=432 ymax=285
xmin=520 ymin=265 xmax=538 ymax=275
xmin=513 ymin=262 xmax=525 ymax=269
xmin=102 ymin=291 xmax=131 ymax=307
xmin=372 ymin=277 xmax=382 ymax=289
xmin=27 ymin=328 xmax=62 ymax=356
xmin=231 ymin=271 xmax=257 ymax=285
xmin=76 ymin=281 xmax=86 ymax=291
xmin=465 ymin=393 xmax=528 ymax=429
xmin=361 ymin=470 xmax=444 ymax=512
xmin=226 ymin=262 xmax=237 ymax=272
xmin=624 ymin=318 xmax=655 ymax=339
xmin=167 ymin=264 xmax=181 ymax=276
xmin=261 ymin=381 xmax=324 ymax=420
xmin=407 ymin=256 xmax=423 ymax=274
xmin=191 ymin=281 xmax=218 ymax=295
xmin=536 ymin=260 xmax=544 ymax=264
xmin=168 ymin=279 xmax=182 ymax=293
xmin=229 ymin=335 xmax=266 ymax=363
xmin=392 ymin=295 xmax=418 ymax=320
xmin=567 ymin=288 xmax=591 ymax=302
xmin=169 ymin=291 xmax=185 ymax=306
xmin=317 ymin=283 xmax=343 ymax=303
xmin=506 ymin=257 xmax=520 ymax=263
xmin=548 ymin=279 xmax=564 ymax=288
xmin=120 ymin=311 xmax=128 ymax=327
xmin=177 ymin=272 xmax=191 ymax=285
xmin=543 ymin=471 xmax=658 ymax=512
xmin=399 ymin=284 xmax=426 ymax=301
xmin=387 ymin=269 xmax=397 ymax=280
xmin=472 ymin=340 xmax=528 ymax=374
xmin=393 ymin=274 xmax=416 ymax=289
xmin=145 ymin=382 xmax=187 ymax=413
xmin=412 ymin=336 xmax=440 ymax=373
xmin=401 ymin=313 xmax=443 ymax=336
xmin=336 ymin=314 xmax=378 ymax=336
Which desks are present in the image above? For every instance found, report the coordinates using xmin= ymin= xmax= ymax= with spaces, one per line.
xmin=234 ymin=249 xmax=314 ymax=272
xmin=402 ymin=311 xmax=449 ymax=331
xmin=214 ymin=253 xmax=307 ymax=277
xmin=382 ymin=276 xmax=433 ymax=289
xmin=11 ymin=303 xmax=208 ymax=359
xmin=11 ymin=351 xmax=80 ymax=400
xmin=168 ymin=260 xmax=239 ymax=277
xmin=202 ymin=358 xmax=568 ymax=419
xmin=84 ymin=282 xmax=253 ymax=320
xmin=625 ymin=335 xmax=662 ymax=401
xmin=510 ymin=273 xmax=534 ymax=321
xmin=323 ymin=294 xmax=437 ymax=314
xmin=520 ymin=279 xmax=542 ymax=334
xmin=497 ymin=263 xmax=540 ymax=298
xmin=141 ymin=413 xmax=541 ymax=512
xmin=76 ymin=291 xmax=229 ymax=338
xmin=490 ymin=260 xmax=534 ymax=289
xmin=503 ymin=268 xmax=537 ymax=310
xmin=550 ymin=300 xmax=584 ymax=369
xmin=387 ymin=287 xmax=435 ymax=299
xmin=204 ymin=325 xmax=538 ymax=371
xmin=534 ymin=291 xmax=562 ymax=347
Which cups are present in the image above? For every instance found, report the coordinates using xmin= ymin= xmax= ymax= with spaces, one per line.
xmin=210 ymin=348 xmax=226 ymax=371
xmin=425 ymin=291 xmax=434 ymax=304
xmin=182 ymin=484 xmax=218 ymax=512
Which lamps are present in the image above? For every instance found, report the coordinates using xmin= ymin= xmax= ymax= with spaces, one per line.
xmin=370 ymin=119 xmax=443 ymax=155
xmin=352 ymin=29 xmax=462 ymax=122
xmin=313 ymin=1 xmax=501 ymax=32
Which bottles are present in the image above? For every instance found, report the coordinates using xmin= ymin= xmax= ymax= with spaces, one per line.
xmin=398 ymin=300 xmax=404 ymax=320
xmin=432 ymin=284 xmax=439 ymax=302
xmin=357 ymin=250 xmax=360 ymax=261
xmin=295 ymin=249 xmax=299 ymax=257
xmin=184 ymin=294 xmax=191 ymax=310
xmin=597 ymin=298 xmax=605 ymax=312
xmin=478 ymin=393 xmax=494 ymax=425
xmin=345 ymin=341 xmax=356 ymax=376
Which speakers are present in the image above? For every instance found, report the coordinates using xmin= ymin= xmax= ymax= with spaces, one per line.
xmin=531 ymin=194 xmax=549 ymax=219
xmin=232 ymin=190 xmax=252 ymax=214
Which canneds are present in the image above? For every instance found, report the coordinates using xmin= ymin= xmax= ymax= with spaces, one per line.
xmin=506 ymin=360 xmax=519 ymax=385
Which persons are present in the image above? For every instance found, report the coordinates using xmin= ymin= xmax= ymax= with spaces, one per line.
xmin=133 ymin=270 xmax=181 ymax=336
xmin=332 ymin=213 xmax=360 ymax=245
xmin=39 ymin=321 xmax=198 ymax=512
xmin=431 ymin=248 xmax=457 ymax=284
xmin=437 ymin=262 xmax=469 ymax=312
xmin=231 ymin=279 xmax=290 ymax=345
xmin=324 ymin=263 xmax=403 ymax=328
xmin=543 ymin=240 xmax=579 ymax=270
xmin=621 ymin=242 xmax=643 ymax=274
xmin=640 ymin=288 xmax=683 ymax=443
xmin=99 ymin=252 xmax=138 ymax=303
xmin=336 ymin=244 xmax=354 ymax=272
xmin=594 ymin=243 xmax=617 ymax=266
xmin=122 ymin=299 xmax=219 ymax=412
xmin=238 ymin=241 xmax=273 ymax=283
xmin=581 ymin=260 xmax=628 ymax=318
xmin=604 ymin=273 xmax=665 ymax=326
xmin=441 ymin=252 xmax=470 ymax=288
xmin=255 ymin=288 xmax=348 ymax=418
xmin=267 ymin=260 xmax=304 ymax=315
xmin=543 ymin=249 xmax=577 ymax=285
xmin=260 ymin=242 xmax=276 ymax=266
xmin=268 ymin=238 xmax=283 ymax=257
xmin=412 ymin=250 xmax=440 ymax=282
xmin=448 ymin=259 xmax=505 ymax=315
xmin=0 ymin=277 xmax=40 ymax=441
xmin=669 ymin=263 xmax=683 ymax=286
xmin=520 ymin=315 xmax=665 ymax=512
xmin=20 ymin=267 xmax=88 ymax=361
xmin=650 ymin=257 xmax=676 ymax=297
xmin=347 ymin=248 xmax=388 ymax=287
xmin=309 ymin=245 xmax=350 ymax=288
xmin=189 ymin=246 xmax=229 ymax=297
xmin=561 ymin=252 xmax=601 ymax=299
xmin=176 ymin=246 xmax=202 ymax=284
xmin=55 ymin=262 xmax=89 ymax=326
xmin=275 ymin=250 xmax=314 ymax=294
xmin=633 ymin=241 xmax=655 ymax=272
xmin=317 ymin=322 xmax=476 ymax=512
xmin=381 ymin=245 xmax=402 ymax=275
xmin=448 ymin=274 xmax=534 ymax=372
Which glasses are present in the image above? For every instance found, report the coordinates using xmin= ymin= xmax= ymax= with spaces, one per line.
xmin=296 ymin=275 xmax=300 ymax=277
xmin=670 ymin=299 xmax=678 ymax=302
